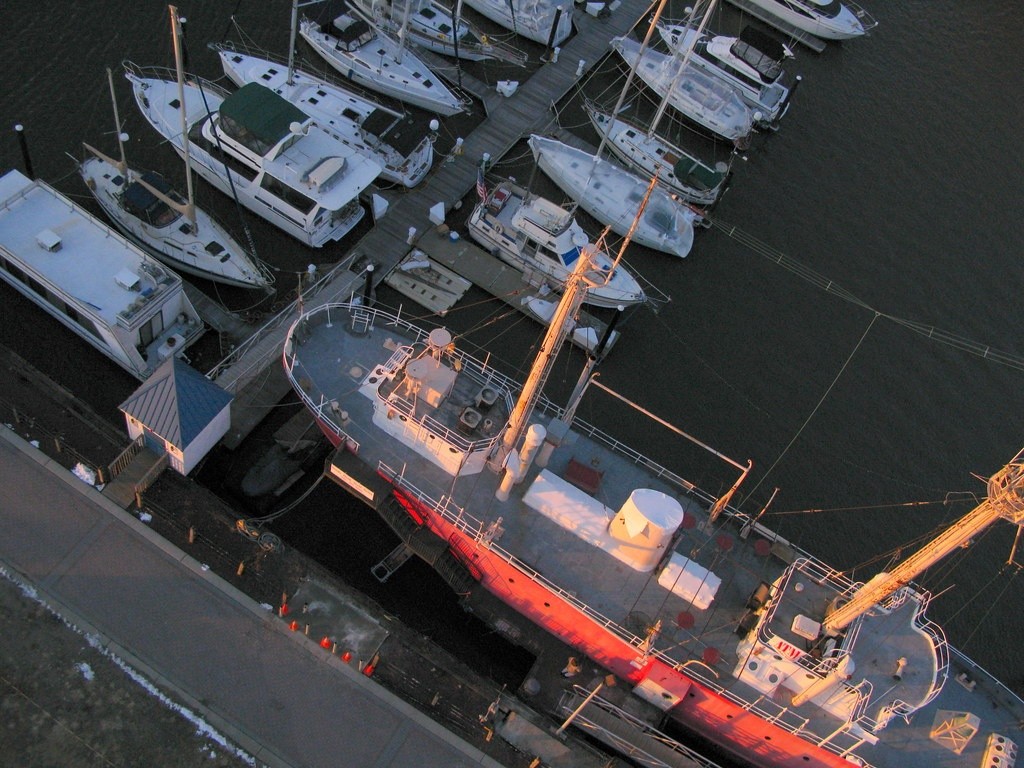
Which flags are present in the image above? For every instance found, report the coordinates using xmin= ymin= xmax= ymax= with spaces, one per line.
xmin=477 ymin=170 xmax=489 ymax=204
xmin=769 ymin=635 xmax=806 ymax=661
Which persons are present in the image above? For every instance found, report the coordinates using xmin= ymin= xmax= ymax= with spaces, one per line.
xmin=562 ymin=656 xmax=582 ymax=677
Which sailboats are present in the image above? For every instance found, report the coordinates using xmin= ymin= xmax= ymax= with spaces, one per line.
xmin=205 ymin=0 xmax=440 ymax=191
xmin=74 ymin=4 xmax=279 ymax=291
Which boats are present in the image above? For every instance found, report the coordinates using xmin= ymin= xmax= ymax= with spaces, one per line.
xmin=719 ymin=0 xmax=879 ymax=40
xmin=0 ymin=167 xmax=208 ymax=383
xmin=119 ymin=59 xmax=388 ymax=251
xmin=281 ymin=162 xmax=1023 ymax=768
xmin=300 ymin=1 xmax=803 ymax=316
